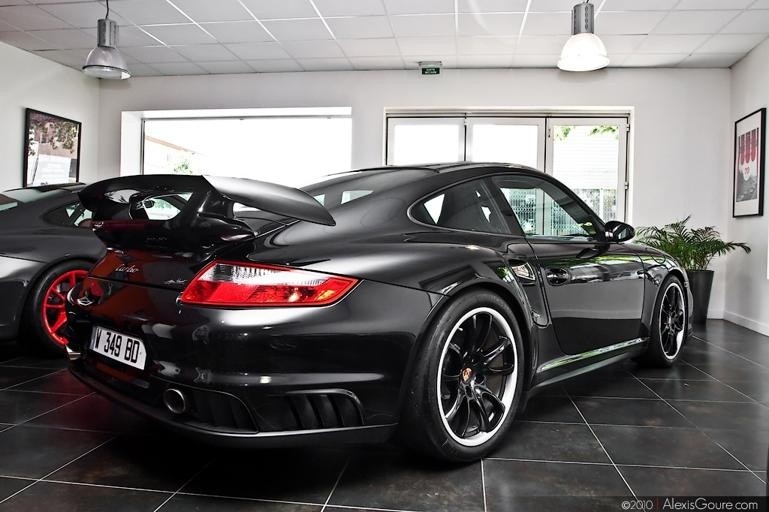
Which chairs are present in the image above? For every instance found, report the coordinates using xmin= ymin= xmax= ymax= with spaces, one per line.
xmin=437 ymin=184 xmax=500 ymax=232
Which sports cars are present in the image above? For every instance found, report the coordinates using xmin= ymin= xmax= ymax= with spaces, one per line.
xmin=0 ymin=179 xmax=217 ymax=367
xmin=62 ymin=155 xmax=702 ymax=469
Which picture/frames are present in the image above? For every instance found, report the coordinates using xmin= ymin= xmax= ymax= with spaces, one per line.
xmin=733 ymin=108 xmax=766 ymax=217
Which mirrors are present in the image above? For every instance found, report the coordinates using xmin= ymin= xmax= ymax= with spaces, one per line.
xmin=23 ymin=108 xmax=81 ymax=189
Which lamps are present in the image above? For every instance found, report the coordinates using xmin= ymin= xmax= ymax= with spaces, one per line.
xmin=82 ymin=0 xmax=132 ymax=80
xmin=557 ymin=0 xmax=609 ymax=71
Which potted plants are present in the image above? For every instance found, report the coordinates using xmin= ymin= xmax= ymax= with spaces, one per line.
xmin=632 ymin=215 xmax=751 ymax=324
